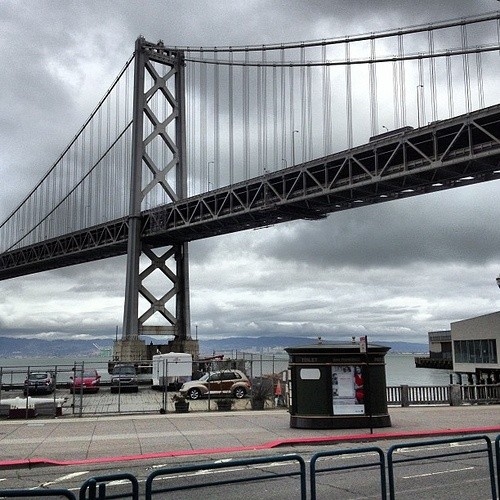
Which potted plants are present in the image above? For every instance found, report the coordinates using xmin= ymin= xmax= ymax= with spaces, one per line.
xmin=215 ymin=398 xmax=236 ymax=410
xmin=248 ymin=377 xmax=274 ymax=410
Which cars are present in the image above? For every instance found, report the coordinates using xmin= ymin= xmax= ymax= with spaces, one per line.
xmin=69 ymin=369 xmax=101 ymax=394
xmin=179 ymin=370 xmax=253 ymax=400
xmin=24 ymin=372 xmax=57 ymax=395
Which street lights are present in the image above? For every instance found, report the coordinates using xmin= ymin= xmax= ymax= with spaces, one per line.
xmin=416 ymin=84 xmax=424 ymax=127
xmin=209 ymin=182 xmax=213 ymax=190
xmin=292 ymin=130 xmax=298 ymax=166
xmin=282 ymin=158 xmax=287 ymax=168
xmin=208 ymin=161 xmax=214 ymax=191
xmin=383 ymin=126 xmax=388 ymax=132
xmin=18 ymin=202 xmax=152 ymax=248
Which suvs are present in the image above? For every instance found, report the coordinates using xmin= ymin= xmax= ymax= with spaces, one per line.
xmin=109 ymin=364 xmax=139 ymax=394
